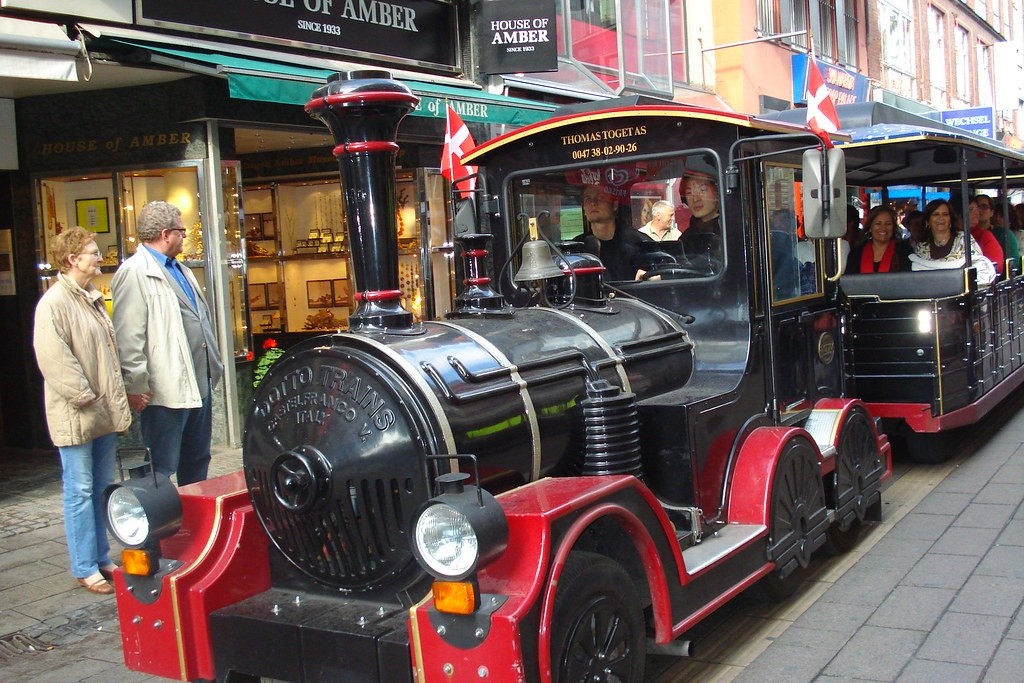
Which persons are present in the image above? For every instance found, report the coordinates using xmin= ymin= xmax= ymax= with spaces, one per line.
xmin=770 ymin=208 xmax=815 ymax=297
xmin=635 ymin=154 xmax=724 ymax=282
xmin=841 ymin=194 xmax=1024 ymax=290
xmin=565 ymin=171 xmax=649 ymax=285
xmin=638 ymin=199 xmax=683 ymax=241
xmin=110 ymin=199 xmax=223 ymax=489
xmin=31 ymin=226 xmax=133 ymax=596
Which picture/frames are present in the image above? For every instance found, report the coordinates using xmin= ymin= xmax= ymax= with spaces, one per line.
xmin=244 ymin=213 xmax=261 ymax=241
xmin=266 ymin=281 xmax=286 ymax=309
xmin=247 ymin=283 xmax=266 ymax=311
xmin=332 ymin=279 xmax=348 ymax=307
xmin=261 ymin=212 xmax=275 ymax=240
xmin=306 ymin=279 xmax=333 ymax=308
xmin=75 ymin=197 xmax=110 ymax=233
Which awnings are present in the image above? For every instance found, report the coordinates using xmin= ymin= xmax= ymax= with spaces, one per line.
xmin=0 ymin=14 xmax=82 ymax=83
xmin=71 ymin=20 xmax=561 ymax=126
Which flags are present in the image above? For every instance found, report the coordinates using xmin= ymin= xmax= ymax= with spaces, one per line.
xmin=805 ymin=55 xmax=840 ymax=149
xmin=440 ymin=106 xmax=480 ymax=200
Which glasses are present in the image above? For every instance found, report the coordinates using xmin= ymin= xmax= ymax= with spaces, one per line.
xmin=978 ymin=205 xmax=992 ymax=209
xmin=75 ymin=251 xmax=102 ymax=257
xmin=159 ymin=228 xmax=186 ymax=236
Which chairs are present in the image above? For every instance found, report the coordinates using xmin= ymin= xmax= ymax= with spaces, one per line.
xmin=768 ymin=230 xmax=797 ymax=301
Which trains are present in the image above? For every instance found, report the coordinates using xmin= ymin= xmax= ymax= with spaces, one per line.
xmin=98 ymin=68 xmax=1024 ymax=682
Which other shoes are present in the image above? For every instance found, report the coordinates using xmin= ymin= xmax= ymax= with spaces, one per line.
xmin=99 ymin=566 xmax=113 ymax=580
xmin=78 ymin=578 xmax=114 ymax=593
